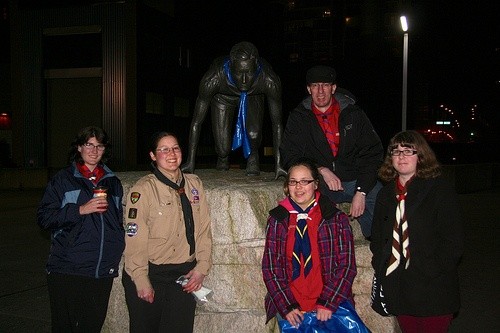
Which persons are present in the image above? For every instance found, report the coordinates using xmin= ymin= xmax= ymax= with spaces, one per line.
xmin=121 ymin=125 xmax=213 ymax=333
xmin=35 ymin=125 xmax=125 ymax=333
xmin=261 ymin=155 xmax=372 ymax=333
xmin=370 ymin=130 xmax=462 ymax=333
xmin=180 ymin=42 xmax=288 ymax=181
xmin=275 ymin=63 xmax=385 ymax=241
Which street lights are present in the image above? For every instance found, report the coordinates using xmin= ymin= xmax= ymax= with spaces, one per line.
xmin=399 ymin=15 xmax=409 ymax=133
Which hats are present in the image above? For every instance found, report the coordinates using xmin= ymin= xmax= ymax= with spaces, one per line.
xmin=306 ymin=65 xmax=336 ymax=83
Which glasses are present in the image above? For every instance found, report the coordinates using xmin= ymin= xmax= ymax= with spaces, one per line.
xmin=287 ymin=179 xmax=314 ymax=186
xmin=390 ymin=149 xmax=419 ymax=157
xmin=158 ymin=148 xmax=179 ymax=152
xmin=82 ymin=142 xmax=104 ymax=149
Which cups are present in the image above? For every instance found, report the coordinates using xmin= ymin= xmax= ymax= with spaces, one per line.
xmin=93 ymin=190 xmax=107 ymax=212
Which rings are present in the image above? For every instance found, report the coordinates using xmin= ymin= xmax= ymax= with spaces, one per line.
xmin=195 ymin=285 xmax=199 ymax=287
xmin=96 ymin=200 xmax=99 ymax=203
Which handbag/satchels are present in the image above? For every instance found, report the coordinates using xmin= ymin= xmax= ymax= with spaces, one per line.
xmin=280 ymin=307 xmax=370 ymax=333
xmin=370 ymin=274 xmax=389 ymax=316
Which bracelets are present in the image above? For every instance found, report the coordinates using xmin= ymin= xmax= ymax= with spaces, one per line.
xmin=356 ymin=190 xmax=366 ymax=196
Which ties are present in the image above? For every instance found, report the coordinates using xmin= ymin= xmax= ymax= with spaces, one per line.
xmin=153 ymin=167 xmax=195 ymax=255
xmin=386 ymin=177 xmax=413 ymax=275
xmin=321 ymin=113 xmax=337 ymax=157
xmin=288 ymin=197 xmax=316 ymax=280
